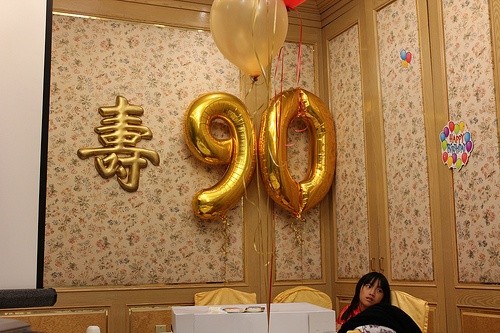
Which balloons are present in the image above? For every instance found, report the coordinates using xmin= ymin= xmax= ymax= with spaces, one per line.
xmin=209 ymin=0 xmax=305 ymax=81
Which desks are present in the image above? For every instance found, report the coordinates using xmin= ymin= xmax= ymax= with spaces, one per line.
xmin=171 ymin=303 xmax=336 ymax=333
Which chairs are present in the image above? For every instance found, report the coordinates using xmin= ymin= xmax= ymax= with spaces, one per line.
xmin=389 ymin=289 xmax=430 ymax=333
xmin=272 ymin=286 xmax=332 ymax=310
xmin=194 ymin=288 xmax=258 ymax=305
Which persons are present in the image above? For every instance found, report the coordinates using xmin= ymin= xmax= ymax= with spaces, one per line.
xmin=336 ymin=272 xmax=390 ymax=333
